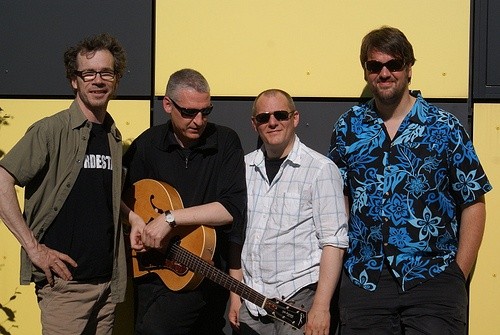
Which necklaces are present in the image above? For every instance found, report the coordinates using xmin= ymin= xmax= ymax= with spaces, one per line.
xmin=175 ymin=135 xmax=204 ymax=169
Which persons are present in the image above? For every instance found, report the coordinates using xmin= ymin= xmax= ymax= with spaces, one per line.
xmin=0 ymin=33 xmax=128 ymax=335
xmin=228 ymin=89 xmax=349 ymax=335
xmin=327 ymin=26 xmax=493 ymax=335
xmin=122 ymin=68 xmax=247 ymax=335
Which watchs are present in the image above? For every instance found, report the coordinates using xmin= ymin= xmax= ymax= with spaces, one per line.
xmin=164 ymin=210 xmax=176 ymax=228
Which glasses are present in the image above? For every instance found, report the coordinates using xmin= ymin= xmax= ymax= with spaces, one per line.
xmin=73 ymin=69 xmax=119 ymax=82
xmin=364 ymin=58 xmax=406 ymax=73
xmin=253 ymin=110 xmax=294 ymax=123
xmin=169 ymin=97 xmax=214 ymax=119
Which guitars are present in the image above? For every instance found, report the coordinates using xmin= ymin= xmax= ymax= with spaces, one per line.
xmin=122 ymin=179 xmax=309 ymax=333
